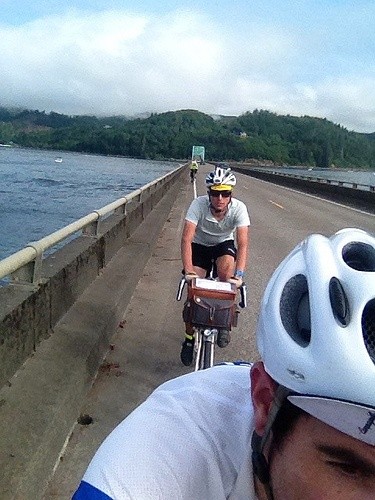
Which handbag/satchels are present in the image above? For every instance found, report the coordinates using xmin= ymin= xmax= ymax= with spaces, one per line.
xmin=189 ymin=277 xmax=239 ymax=329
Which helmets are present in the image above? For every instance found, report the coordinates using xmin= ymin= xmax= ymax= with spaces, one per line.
xmin=205 ymin=167 xmax=237 ymax=190
xmin=254 ymin=226 xmax=375 ymax=449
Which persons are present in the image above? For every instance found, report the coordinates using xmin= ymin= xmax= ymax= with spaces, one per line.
xmin=190 ymin=162 xmax=198 ymax=183
xmin=180 ymin=167 xmax=250 ymax=367
xmin=73 ymin=228 xmax=375 ymax=500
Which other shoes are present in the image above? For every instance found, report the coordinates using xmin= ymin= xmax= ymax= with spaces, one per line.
xmin=180 ymin=336 xmax=195 ymax=368
xmin=217 ymin=330 xmax=231 ymax=348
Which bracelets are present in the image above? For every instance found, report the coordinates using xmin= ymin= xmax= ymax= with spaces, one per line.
xmin=235 ymin=270 xmax=244 ymax=277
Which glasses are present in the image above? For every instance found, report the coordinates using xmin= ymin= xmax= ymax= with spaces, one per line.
xmin=211 ymin=190 xmax=232 ymax=198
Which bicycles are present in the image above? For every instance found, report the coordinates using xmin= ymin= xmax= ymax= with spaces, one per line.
xmin=176 ymin=259 xmax=247 ymax=371
xmin=192 ymin=170 xmax=196 ymax=178
